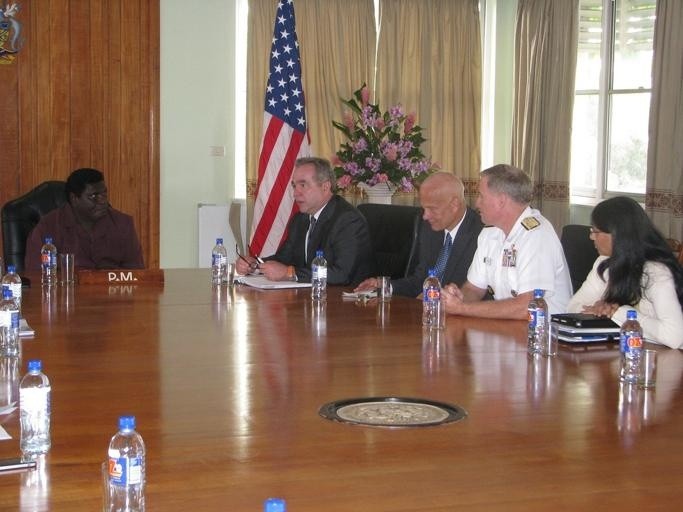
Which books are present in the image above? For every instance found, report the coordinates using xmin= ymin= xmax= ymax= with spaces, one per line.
xmin=234 ymin=274 xmax=312 ymax=289
xmin=551 ymin=313 xmax=622 ymax=345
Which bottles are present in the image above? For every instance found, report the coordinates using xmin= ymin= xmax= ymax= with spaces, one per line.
xmin=265 ymin=495 xmax=292 ymax=512
xmin=107 ymin=414 xmax=146 ymax=512
xmin=423 ymin=270 xmax=441 ymax=328
xmin=212 ymin=239 xmax=228 ymax=285
xmin=529 ymin=289 xmax=547 ymax=358
xmin=0 ymin=291 xmax=18 ymax=358
xmin=311 ymin=249 xmax=328 ymax=300
xmin=41 ymin=236 xmax=58 ymax=284
xmin=1 ymin=266 xmax=21 ymax=312
xmin=620 ymin=310 xmax=643 ymax=384
xmin=19 ymin=358 xmax=52 ymax=456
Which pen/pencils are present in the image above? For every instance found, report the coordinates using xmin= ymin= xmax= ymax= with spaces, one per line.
xmin=254 ymin=254 xmax=264 ymax=265
xmin=370 ymin=288 xmax=378 ymax=292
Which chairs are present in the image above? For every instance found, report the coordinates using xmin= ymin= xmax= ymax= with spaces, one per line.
xmin=560 ymin=224 xmax=600 ymax=296
xmin=2 ymin=181 xmax=112 ymax=272
xmin=358 ymin=205 xmax=424 ymax=279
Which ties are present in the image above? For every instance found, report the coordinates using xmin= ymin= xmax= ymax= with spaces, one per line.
xmin=429 ymin=232 xmax=454 ymax=280
xmin=308 ymin=214 xmax=317 ymax=231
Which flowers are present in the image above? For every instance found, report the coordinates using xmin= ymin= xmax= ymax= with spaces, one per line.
xmin=331 ymin=81 xmax=442 ymax=198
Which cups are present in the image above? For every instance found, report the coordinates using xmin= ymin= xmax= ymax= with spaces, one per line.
xmin=221 ymin=262 xmax=236 ymax=288
xmin=541 ymin=319 xmax=559 ymax=359
xmin=57 ymin=253 xmax=76 ymax=290
xmin=430 ymin=297 xmax=447 ymax=332
xmin=376 ymin=275 xmax=394 ymax=303
xmin=636 ymin=348 xmax=658 ymax=391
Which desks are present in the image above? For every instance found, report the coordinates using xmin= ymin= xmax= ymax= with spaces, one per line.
xmin=0 ymin=267 xmax=683 ymax=512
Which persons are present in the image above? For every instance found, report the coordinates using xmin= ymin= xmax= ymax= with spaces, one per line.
xmin=354 ymin=172 xmax=483 ymax=297
xmin=234 ymin=158 xmax=374 ymax=284
xmin=567 ymin=196 xmax=683 ymax=350
xmin=441 ymin=165 xmax=575 ymax=320
xmin=25 ymin=168 xmax=145 ymax=271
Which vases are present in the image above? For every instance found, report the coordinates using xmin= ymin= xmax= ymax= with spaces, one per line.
xmin=355 ymin=180 xmax=402 ymax=205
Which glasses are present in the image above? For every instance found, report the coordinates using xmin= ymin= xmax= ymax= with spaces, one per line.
xmin=588 ymin=226 xmax=606 ymax=235
xmin=235 ymin=242 xmax=262 ymax=270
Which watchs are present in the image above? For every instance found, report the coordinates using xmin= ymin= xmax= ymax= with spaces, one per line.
xmin=287 ymin=265 xmax=295 ymax=282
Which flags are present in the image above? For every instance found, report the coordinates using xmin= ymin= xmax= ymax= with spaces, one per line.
xmin=248 ymin=1 xmax=313 ymax=260
